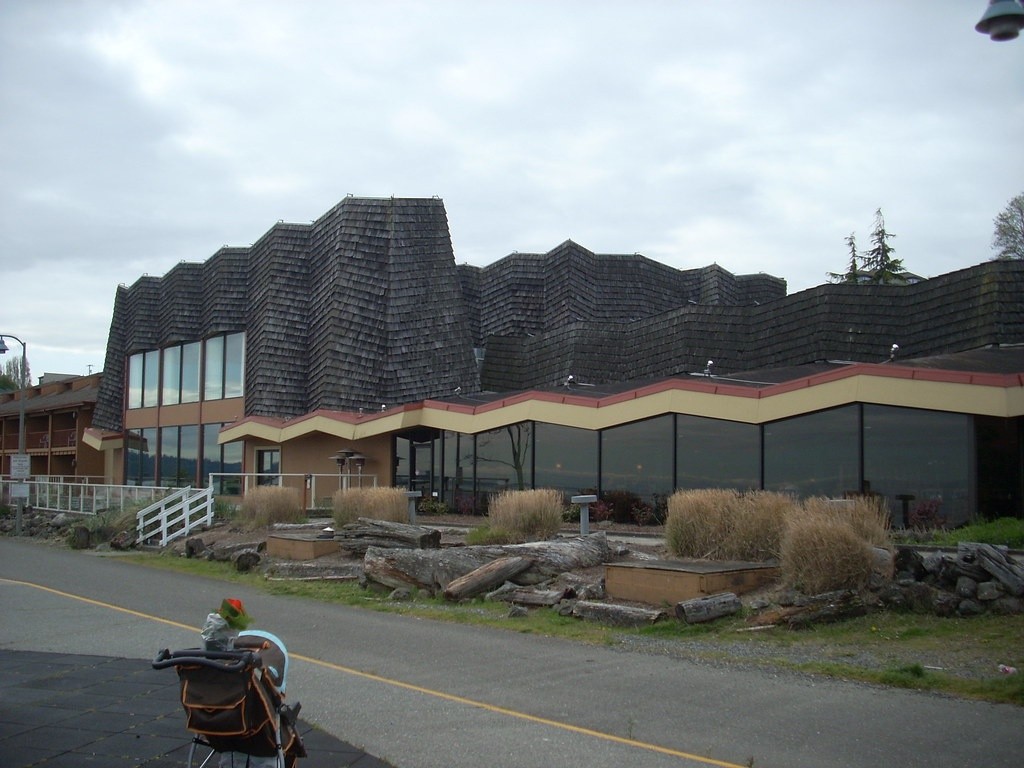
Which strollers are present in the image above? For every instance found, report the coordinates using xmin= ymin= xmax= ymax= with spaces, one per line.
xmin=151 ymin=629 xmax=308 ymax=768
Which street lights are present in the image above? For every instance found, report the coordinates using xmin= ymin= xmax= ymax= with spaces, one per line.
xmin=0 ymin=334 xmax=27 ymax=534
